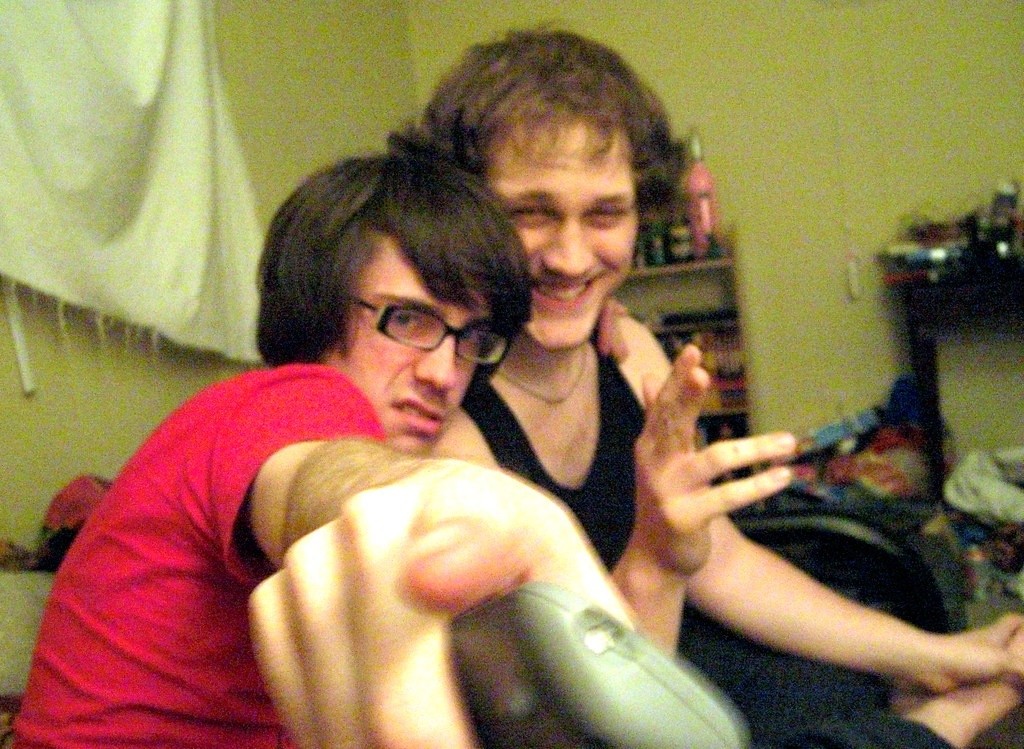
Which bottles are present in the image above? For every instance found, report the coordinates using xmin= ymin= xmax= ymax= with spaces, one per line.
xmin=634 ymin=128 xmax=719 ymax=270
xmin=957 ymin=527 xmax=997 ymax=635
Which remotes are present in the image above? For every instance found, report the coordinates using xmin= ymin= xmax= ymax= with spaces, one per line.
xmin=450 ymin=577 xmax=756 ymax=749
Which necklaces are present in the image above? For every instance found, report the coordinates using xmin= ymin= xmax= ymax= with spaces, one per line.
xmin=496 ymin=350 xmax=586 ymax=403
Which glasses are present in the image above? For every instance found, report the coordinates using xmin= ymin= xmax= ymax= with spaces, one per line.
xmin=330 ymin=291 xmax=511 ymax=365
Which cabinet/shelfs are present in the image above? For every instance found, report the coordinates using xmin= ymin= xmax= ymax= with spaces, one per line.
xmin=612 ymin=219 xmax=762 ymax=518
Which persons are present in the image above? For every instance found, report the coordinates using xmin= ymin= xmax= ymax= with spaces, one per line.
xmin=12 ymin=29 xmax=1023 ymax=749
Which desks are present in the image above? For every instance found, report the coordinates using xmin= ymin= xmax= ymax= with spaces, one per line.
xmin=886 ymin=260 xmax=1024 ymax=497
